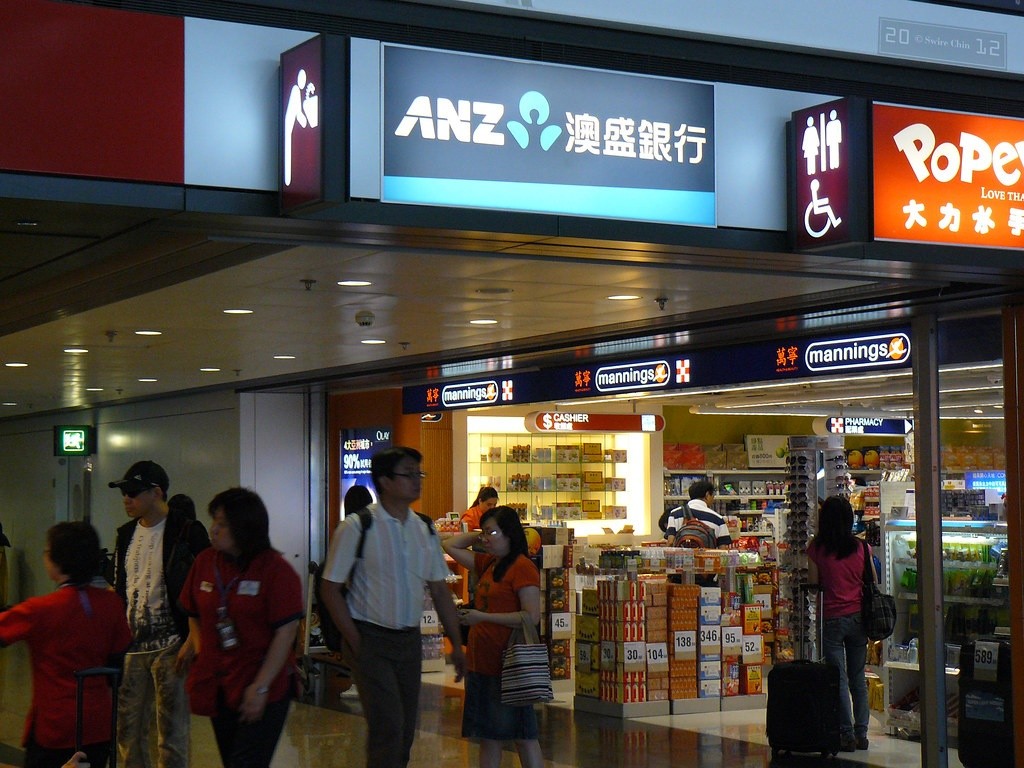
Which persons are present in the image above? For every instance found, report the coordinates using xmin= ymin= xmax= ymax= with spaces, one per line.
xmin=0 ymin=519 xmax=132 ymax=768
xmin=442 ymin=506 xmax=543 ymax=768
xmin=107 ymin=460 xmax=213 ymax=768
xmin=344 ymin=485 xmax=373 ymax=515
xmin=462 ymin=487 xmax=498 ymax=532
xmin=807 ymin=495 xmax=879 ymax=751
xmin=180 ymin=488 xmax=305 ymax=768
xmin=321 ymin=446 xmax=466 ymax=768
xmin=666 ymin=481 xmax=731 ymax=587
xmin=61 ymin=752 xmax=90 ymax=768
xmin=0 ymin=523 xmax=10 ymax=611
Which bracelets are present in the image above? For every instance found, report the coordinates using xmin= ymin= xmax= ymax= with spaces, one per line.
xmin=254 ymin=684 xmax=269 ymax=694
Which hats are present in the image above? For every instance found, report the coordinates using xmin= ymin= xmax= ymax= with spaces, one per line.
xmin=108 ymin=461 xmax=170 ymax=493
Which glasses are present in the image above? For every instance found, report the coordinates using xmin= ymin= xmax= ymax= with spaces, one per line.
xmin=394 ymin=471 xmax=426 ymax=479
xmin=785 ymin=455 xmax=853 ymax=642
xmin=477 ymin=529 xmax=498 ymax=539
xmin=121 ymin=487 xmax=154 ymax=497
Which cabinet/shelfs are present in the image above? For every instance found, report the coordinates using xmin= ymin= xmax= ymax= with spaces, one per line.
xmin=442 ymin=558 xmax=467 ymax=655
xmin=664 ymin=469 xmax=882 ymax=537
xmin=467 ymin=431 xmax=629 ymax=523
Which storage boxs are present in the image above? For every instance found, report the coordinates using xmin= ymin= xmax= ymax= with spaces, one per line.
xmin=520 ymin=523 xmax=884 ymax=718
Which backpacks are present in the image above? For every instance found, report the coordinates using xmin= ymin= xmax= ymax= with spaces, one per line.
xmin=673 ymin=503 xmax=717 ymax=548
xmin=313 ymin=506 xmax=435 ymax=654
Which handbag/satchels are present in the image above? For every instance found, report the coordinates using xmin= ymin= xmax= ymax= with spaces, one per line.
xmin=500 ymin=610 xmax=554 ymax=707
xmin=456 ymin=604 xmax=474 ymax=646
xmin=861 ymin=539 xmax=896 ymax=640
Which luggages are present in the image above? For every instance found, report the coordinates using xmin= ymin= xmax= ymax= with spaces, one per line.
xmin=958 ymin=638 xmax=1016 ymax=768
xmin=766 ymin=583 xmax=842 ymax=758
